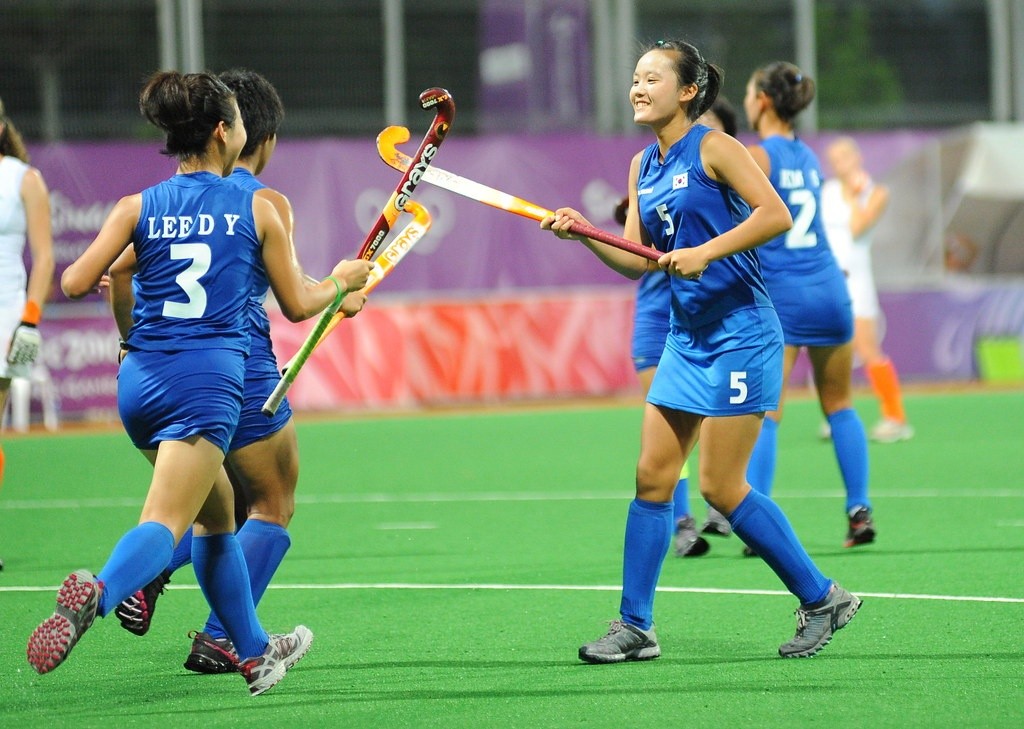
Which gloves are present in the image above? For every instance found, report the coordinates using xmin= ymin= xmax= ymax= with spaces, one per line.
xmin=9 ymin=324 xmax=41 ymax=366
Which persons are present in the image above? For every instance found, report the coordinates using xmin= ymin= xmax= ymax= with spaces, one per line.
xmin=535 ymin=39 xmax=865 ymax=666
xmin=27 ymin=68 xmax=317 ymax=699
xmin=738 ymin=58 xmax=873 ymax=551
xmin=103 ymin=66 xmax=370 ymax=677
xmin=627 ymin=93 xmax=745 ymax=559
xmin=816 ymin=135 xmax=914 ymax=448
xmin=0 ymin=98 xmax=57 ymax=574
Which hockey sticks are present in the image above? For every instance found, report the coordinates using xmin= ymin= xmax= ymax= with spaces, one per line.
xmin=261 ymin=88 xmax=703 ymax=419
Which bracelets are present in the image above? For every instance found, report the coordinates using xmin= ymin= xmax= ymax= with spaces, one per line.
xmin=320 ymin=275 xmax=341 ymax=308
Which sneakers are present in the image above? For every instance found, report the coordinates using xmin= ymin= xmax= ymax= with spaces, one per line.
xmin=114 ymin=570 xmax=169 ymax=634
xmin=240 ymin=625 xmax=314 ymax=696
xmin=675 ymin=515 xmax=708 ymax=557
xmin=845 ymin=509 xmax=876 ymax=548
xmin=703 ymin=505 xmax=732 ymax=539
xmin=579 ymin=619 xmax=662 ymax=665
xmin=26 ymin=570 xmax=101 ymax=674
xmin=873 ymin=417 xmax=914 ymax=443
xmin=184 ymin=630 xmax=245 ymax=673
xmin=780 ymin=584 xmax=863 ymax=658
